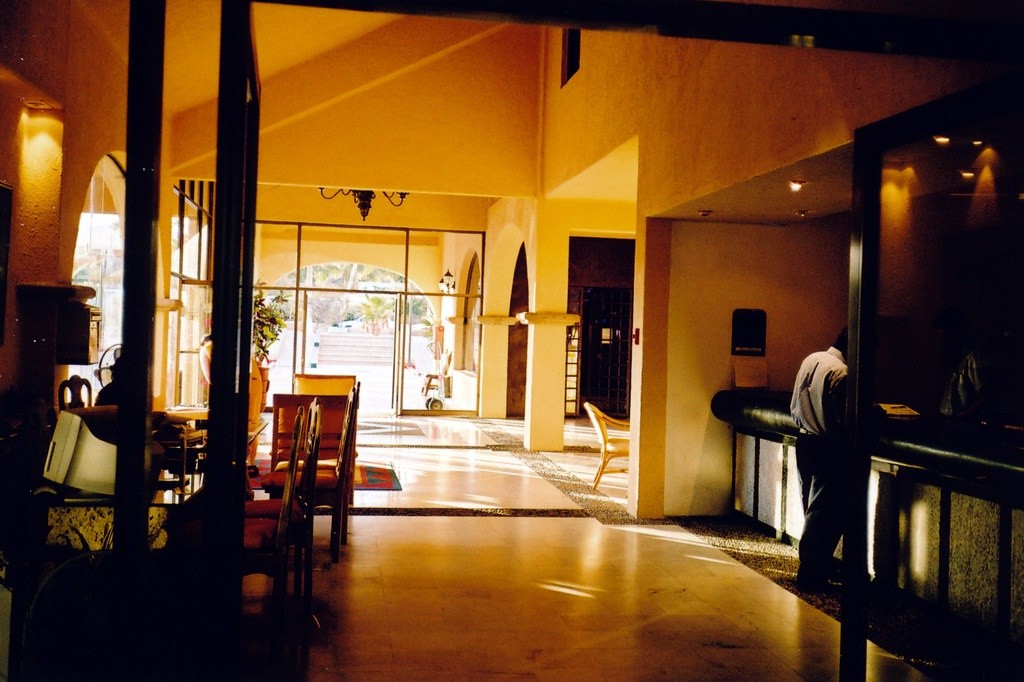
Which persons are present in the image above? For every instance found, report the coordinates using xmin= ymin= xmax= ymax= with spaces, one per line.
xmin=787 ymin=324 xmax=851 ymax=593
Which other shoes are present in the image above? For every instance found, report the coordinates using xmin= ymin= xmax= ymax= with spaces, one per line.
xmin=824 ymin=578 xmax=844 ymax=589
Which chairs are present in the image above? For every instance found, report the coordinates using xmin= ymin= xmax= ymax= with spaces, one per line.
xmin=171 ymin=382 xmax=359 ymax=681
xmin=585 ymin=402 xmax=631 ymax=490
xmin=59 ymin=375 xmax=92 ymax=410
xmin=272 ymin=374 xmax=357 ymax=474
xmin=419 ymin=350 xmax=453 ymax=398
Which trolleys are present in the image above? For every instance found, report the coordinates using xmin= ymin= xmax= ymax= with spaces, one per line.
xmin=421 ymin=349 xmax=453 ymax=411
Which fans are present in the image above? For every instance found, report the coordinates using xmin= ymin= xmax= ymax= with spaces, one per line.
xmin=99 ymin=344 xmax=122 ymax=388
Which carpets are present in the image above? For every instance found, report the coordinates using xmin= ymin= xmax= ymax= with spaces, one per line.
xmin=249 ymin=459 xmax=403 ymax=491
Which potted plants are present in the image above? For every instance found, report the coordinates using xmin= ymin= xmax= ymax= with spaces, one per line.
xmin=251 ymin=277 xmax=289 ymax=413
xmin=361 ymin=294 xmax=395 ymax=336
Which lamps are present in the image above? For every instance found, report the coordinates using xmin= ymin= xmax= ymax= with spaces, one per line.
xmin=319 ymin=188 xmax=410 ymax=222
xmin=439 ymin=269 xmax=455 ymax=293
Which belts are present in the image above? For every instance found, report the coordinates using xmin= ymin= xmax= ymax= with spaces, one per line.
xmin=797 ymin=429 xmax=819 ymax=440
